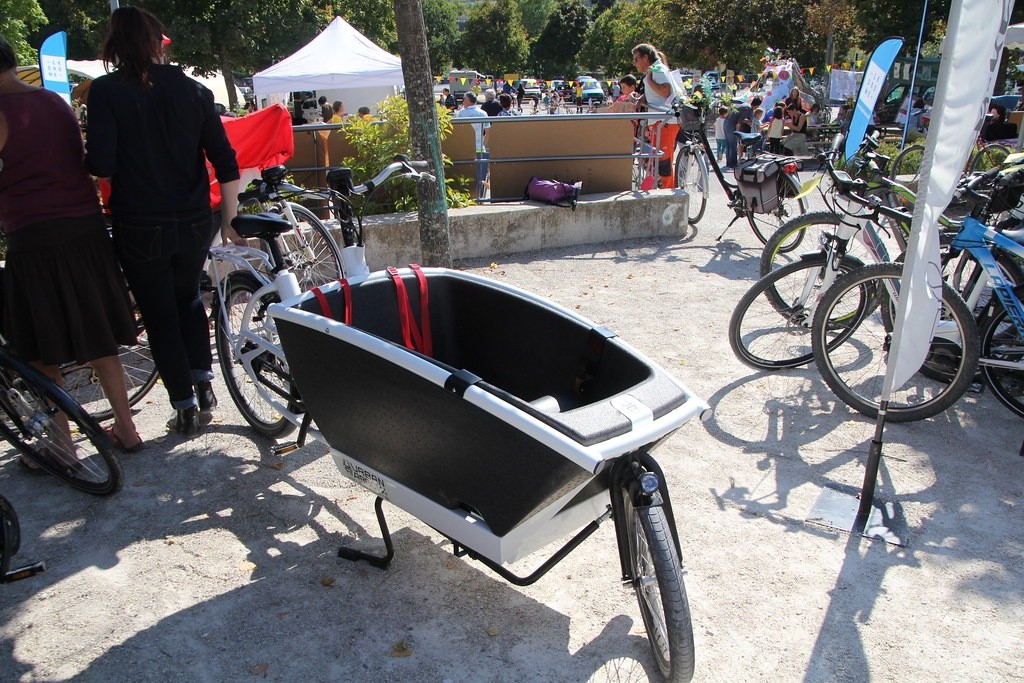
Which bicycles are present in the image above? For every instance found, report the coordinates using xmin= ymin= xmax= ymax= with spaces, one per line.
xmin=726 ymin=126 xmax=1024 ymax=421
xmin=1 ymin=215 xmax=165 ymax=497
xmin=206 ymin=154 xmax=709 ymax=683
xmin=666 ymin=98 xmax=808 ymax=253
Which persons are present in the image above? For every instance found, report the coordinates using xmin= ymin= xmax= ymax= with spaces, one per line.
xmin=440 ymin=44 xmax=706 ymax=205
xmin=289 ymin=92 xmax=379 ymax=124
xmin=0 ymin=41 xmax=143 ymax=474
xmin=84 ymin=7 xmax=242 ymax=438
xmin=715 ymin=80 xmax=936 ymax=172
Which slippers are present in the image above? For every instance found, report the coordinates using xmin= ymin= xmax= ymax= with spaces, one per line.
xmin=20 ymin=447 xmax=82 ymax=473
xmin=103 ymin=424 xmax=143 ymax=453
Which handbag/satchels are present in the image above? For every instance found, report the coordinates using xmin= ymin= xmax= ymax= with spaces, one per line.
xmin=521 ymin=176 xmax=579 ymax=211
xmin=735 ymin=152 xmax=800 ymax=215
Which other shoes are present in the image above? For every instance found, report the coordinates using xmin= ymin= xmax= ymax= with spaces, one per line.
xmin=716 ymin=159 xmax=722 ymax=163
xmin=167 ymin=406 xmax=200 ymax=439
xmin=724 ymin=166 xmax=734 ymax=173
xmin=194 ymin=381 xmax=217 ymax=413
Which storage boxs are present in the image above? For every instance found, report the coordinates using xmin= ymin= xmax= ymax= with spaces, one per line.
xmin=735 ymin=150 xmax=805 ymax=213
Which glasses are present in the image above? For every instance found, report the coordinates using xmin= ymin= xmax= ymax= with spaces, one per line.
xmin=632 ymin=55 xmax=645 ymax=63
xmin=462 ymin=97 xmax=468 ymax=102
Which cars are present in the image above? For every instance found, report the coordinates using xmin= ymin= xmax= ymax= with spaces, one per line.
xmin=497 ymin=79 xmax=565 ymax=100
xmin=554 ymin=84 xmax=572 ymax=101
xmin=571 ymin=76 xmax=604 ymax=104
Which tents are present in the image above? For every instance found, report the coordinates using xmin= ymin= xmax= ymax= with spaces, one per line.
xmin=15 ymin=59 xmax=246 ymax=108
xmin=252 ymin=17 xmax=406 ymax=123
